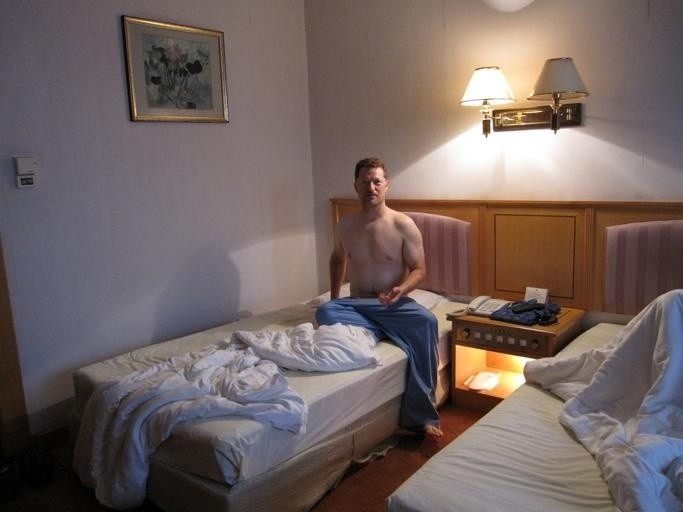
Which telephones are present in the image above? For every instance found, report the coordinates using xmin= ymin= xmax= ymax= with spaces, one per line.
xmin=467 ymin=296 xmax=511 ymax=316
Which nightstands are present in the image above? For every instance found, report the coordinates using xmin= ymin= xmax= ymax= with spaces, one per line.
xmin=447 ymin=301 xmax=586 ymax=414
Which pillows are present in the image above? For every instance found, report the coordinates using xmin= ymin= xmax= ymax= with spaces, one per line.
xmin=303 ymin=279 xmax=451 ymax=314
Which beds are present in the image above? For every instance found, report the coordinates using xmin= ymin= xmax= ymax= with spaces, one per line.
xmin=384 ymin=201 xmax=683 ymax=512
xmin=71 ymin=197 xmax=481 ymax=512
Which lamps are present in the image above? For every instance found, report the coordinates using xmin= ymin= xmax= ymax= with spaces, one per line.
xmin=525 ymin=57 xmax=590 ymax=134
xmin=459 ymin=65 xmax=517 ymax=139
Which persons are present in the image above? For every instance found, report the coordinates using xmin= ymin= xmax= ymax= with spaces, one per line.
xmin=314 ymin=158 xmax=443 ymax=436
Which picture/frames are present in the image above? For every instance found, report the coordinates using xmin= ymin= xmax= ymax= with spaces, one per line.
xmin=121 ymin=14 xmax=230 ymax=123
